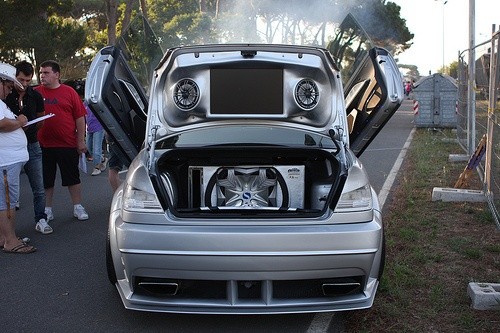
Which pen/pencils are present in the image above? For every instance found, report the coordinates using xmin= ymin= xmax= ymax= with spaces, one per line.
xmin=15 ymin=115 xmax=18 ymax=119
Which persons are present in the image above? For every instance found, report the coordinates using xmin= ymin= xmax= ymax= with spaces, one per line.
xmin=402 ymin=79 xmax=415 ymax=96
xmin=0 ymin=62 xmax=38 ymax=254
xmin=4 ymin=59 xmax=127 ymax=243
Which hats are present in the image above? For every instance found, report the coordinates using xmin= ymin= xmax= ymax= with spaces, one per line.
xmin=0 ymin=63 xmax=24 ymax=90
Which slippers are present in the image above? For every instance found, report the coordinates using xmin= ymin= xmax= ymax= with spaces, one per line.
xmin=0 ymin=236 xmax=31 ymax=248
xmin=2 ymin=241 xmax=38 ymax=254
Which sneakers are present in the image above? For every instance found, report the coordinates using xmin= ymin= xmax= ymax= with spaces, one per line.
xmin=73 ymin=203 xmax=89 ymax=220
xmin=35 ymin=218 xmax=53 ymax=234
xmin=44 ymin=206 xmax=54 ymax=220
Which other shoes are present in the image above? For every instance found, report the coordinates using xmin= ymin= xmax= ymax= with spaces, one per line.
xmin=100 ymin=156 xmax=108 ymax=171
xmin=91 ymin=166 xmax=101 ymax=175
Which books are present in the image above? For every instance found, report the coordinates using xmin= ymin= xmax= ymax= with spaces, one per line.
xmin=22 ymin=113 xmax=55 ymax=127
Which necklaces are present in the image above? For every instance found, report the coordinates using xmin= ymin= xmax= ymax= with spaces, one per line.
xmin=18 ymin=96 xmax=23 ymax=107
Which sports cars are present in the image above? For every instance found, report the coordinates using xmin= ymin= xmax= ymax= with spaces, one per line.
xmin=82 ymin=11 xmax=406 ymax=317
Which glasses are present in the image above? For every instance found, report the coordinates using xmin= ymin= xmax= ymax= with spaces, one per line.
xmin=1 ymin=83 xmax=13 ymax=91
